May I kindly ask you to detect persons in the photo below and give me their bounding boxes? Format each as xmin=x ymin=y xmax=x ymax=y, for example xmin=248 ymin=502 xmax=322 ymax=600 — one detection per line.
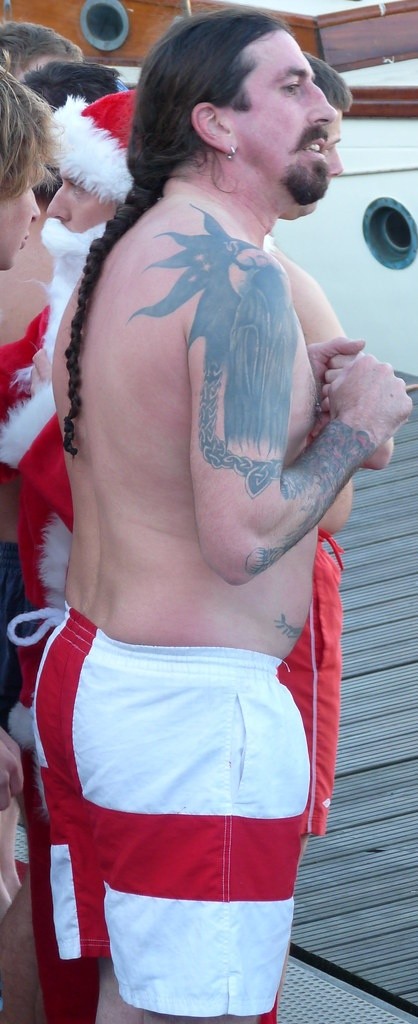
xmin=35 ymin=7 xmax=413 ymax=1024
xmin=0 ymin=23 xmax=396 ymax=1024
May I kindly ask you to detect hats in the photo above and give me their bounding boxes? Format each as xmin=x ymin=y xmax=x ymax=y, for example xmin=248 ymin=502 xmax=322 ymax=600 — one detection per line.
xmin=50 ymin=88 xmax=136 ymax=204
